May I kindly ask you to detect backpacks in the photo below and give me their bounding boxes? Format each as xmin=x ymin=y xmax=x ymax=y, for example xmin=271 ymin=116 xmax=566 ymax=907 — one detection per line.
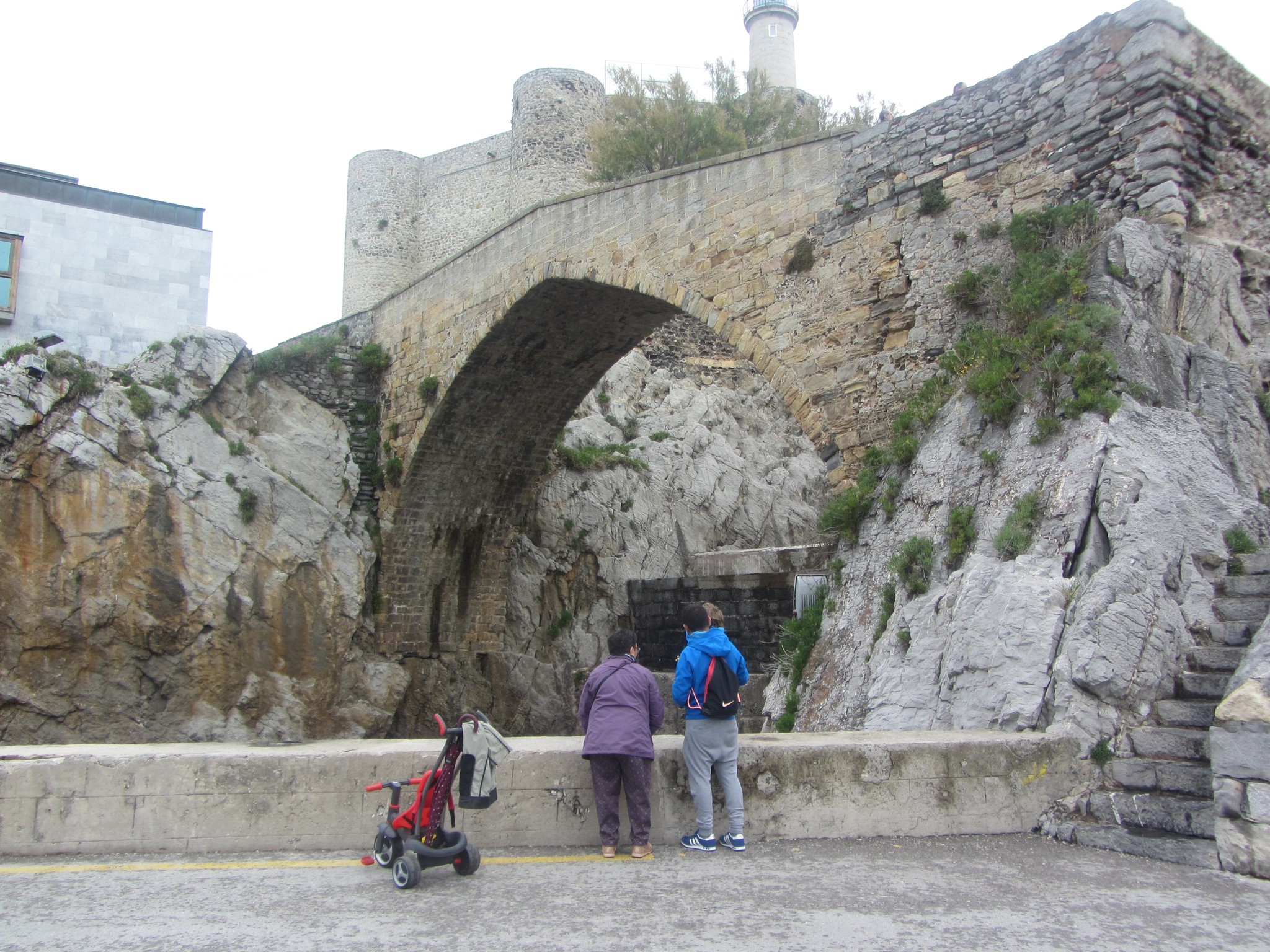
xmin=688 ymin=655 xmax=741 ymax=719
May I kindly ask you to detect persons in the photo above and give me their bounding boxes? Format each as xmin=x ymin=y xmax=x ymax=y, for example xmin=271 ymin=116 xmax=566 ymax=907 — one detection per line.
xmin=578 ymin=628 xmax=664 ymax=858
xmin=672 ymin=603 xmax=750 ymax=851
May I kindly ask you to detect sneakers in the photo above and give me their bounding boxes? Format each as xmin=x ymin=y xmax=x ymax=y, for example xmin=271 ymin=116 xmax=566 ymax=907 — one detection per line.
xmin=602 ymin=845 xmax=616 ymax=857
xmin=631 ymin=842 xmax=652 ymax=857
xmin=680 ymin=829 xmax=716 ymax=851
xmin=720 ymin=831 xmax=746 ymax=851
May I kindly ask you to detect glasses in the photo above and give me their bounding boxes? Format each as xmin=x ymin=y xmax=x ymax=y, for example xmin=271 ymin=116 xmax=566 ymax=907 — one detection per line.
xmin=630 ymin=647 xmax=640 ymax=653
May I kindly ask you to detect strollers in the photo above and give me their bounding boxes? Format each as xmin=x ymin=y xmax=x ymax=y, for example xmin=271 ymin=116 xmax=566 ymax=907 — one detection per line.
xmin=361 ymin=709 xmax=489 ymax=889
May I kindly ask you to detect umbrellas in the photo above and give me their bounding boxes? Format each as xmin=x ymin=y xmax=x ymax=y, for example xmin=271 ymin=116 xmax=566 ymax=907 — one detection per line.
xmin=424 ymin=714 xmax=478 ymax=847
